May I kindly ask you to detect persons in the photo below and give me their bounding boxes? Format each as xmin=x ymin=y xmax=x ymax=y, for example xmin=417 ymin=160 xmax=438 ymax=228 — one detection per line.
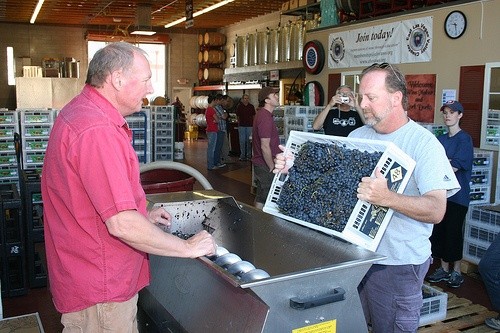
xmin=42 ymin=41 xmax=218 ymax=333
xmin=236 ymin=94 xmax=255 ymax=160
xmin=428 ymin=100 xmax=474 ymax=287
xmin=477 ymin=231 xmax=500 ymax=330
xmin=253 ymin=87 xmax=281 ymax=210
xmin=272 ymin=65 xmax=461 ymax=333
xmin=312 ymin=85 xmax=368 ymax=137
xmin=205 ymin=94 xmax=233 ymax=169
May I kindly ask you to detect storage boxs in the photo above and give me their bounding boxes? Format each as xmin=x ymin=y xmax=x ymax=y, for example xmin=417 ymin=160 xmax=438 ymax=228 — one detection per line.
xmin=271 ymin=105 xmax=326 ymax=145
xmin=417 ymin=285 xmax=448 ymax=327
xmin=261 ymin=130 xmax=415 ymax=253
xmin=413 ymin=122 xmax=500 ymax=265
xmin=125 ymin=104 xmax=175 ymax=163
xmin=1 ymin=109 xmax=61 ymax=195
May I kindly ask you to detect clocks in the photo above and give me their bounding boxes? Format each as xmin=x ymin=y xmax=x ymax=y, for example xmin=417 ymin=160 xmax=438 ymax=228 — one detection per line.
xmin=443 ymin=9 xmax=468 ymax=40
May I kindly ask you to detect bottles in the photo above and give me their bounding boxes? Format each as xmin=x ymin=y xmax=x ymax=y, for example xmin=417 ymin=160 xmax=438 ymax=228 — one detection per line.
xmin=235 ymin=18 xmax=320 ymax=65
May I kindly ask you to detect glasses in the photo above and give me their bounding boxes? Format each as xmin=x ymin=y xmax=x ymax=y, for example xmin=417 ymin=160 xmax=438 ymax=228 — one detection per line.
xmin=372 ymin=62 xmax=402 ymax=83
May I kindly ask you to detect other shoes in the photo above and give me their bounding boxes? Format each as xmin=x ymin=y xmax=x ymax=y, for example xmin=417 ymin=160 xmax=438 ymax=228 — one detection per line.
xmin=217 ymin=163 xmax=225 ymax=167
xmin=247 ymin=157 xmax=251 ymax=162
xmin=223 ymin=160 xmax=231 ymax=164
xmin=239 ymin=157 xmax=246 ymax=161
xmin=210 ymin=165 xmax=218 ymax=169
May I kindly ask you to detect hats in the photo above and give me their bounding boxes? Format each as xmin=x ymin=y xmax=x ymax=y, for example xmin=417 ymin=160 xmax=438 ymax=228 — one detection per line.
xmin=257 ymin=87 xmax=280 ymax=101
xmin=440 ymin=100 xmax=463 ymax=115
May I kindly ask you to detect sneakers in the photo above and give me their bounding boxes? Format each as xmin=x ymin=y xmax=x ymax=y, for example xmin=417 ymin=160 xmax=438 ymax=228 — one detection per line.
xmin=428 ymin=267 xmax=450 ymax=283
xmin=447 ymin=271 xmax=464 ymax=287
xmin=485 ymin=317 xmax=500 ymax=331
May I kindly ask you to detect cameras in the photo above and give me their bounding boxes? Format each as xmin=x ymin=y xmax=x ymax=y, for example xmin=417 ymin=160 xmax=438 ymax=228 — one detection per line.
xmin=338 ymin=97 xmax=349 ymax=104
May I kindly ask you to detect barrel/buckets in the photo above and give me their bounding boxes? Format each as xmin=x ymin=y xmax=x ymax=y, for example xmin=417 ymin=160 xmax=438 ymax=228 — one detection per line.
xmin=188 ymin=95 xmax=213 ymax=127
xmin=65 ymin=58 xmax=79 ymax=78
xmin=197 ymin=32 xmax=225 ymax=82
